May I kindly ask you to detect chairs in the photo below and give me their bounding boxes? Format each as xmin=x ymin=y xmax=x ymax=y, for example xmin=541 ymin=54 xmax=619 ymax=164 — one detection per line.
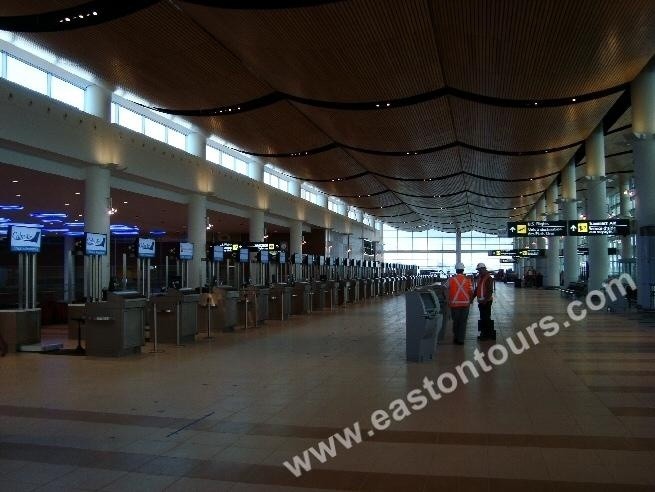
xmin=559 ymin=282 xmax=587 ymax=300
xmin=624 ymin=287 xmax=638 ymax=308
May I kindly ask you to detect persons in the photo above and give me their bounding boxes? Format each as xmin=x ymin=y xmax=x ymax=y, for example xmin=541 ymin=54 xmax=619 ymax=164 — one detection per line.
xmin=470 ymin=263 xmax=496 ymax=340
xmin=444 ymin=262 xmax=473 ymax=344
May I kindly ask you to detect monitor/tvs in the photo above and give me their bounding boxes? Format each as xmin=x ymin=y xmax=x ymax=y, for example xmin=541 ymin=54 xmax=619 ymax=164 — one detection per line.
xmin=307 ymin=254 xmax=313 ymax=264
xmin=294 ymin=253 xmax=301 ymax=264
xmin=279 ymin=252 xmax=286 ymax=264
xmin=213 ymin=245 xmax=224 ymax=262
xmin=179 ymin=242 xmax=194 ymax=260
xmin=239 ymin=248 xmax=249 ymax=263
xmin=9 ymin=225 xmax=43 ymax=254
xmin=319 ymin=255 xmax=412 ymax=270
xmin=260 ymin=250 xmax=269 ymax=263
xmin=136 ymin=237 xmax=157 ymax=258
xmin=85 ymin=232 xmax=107 ymax=255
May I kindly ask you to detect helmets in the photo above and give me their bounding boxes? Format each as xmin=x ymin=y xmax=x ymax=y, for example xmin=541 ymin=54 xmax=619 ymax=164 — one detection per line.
xmin=455 ymin=263 xmax=465 ymax=270
xmin=476 ymin=263 xmax=486 ymax=270
